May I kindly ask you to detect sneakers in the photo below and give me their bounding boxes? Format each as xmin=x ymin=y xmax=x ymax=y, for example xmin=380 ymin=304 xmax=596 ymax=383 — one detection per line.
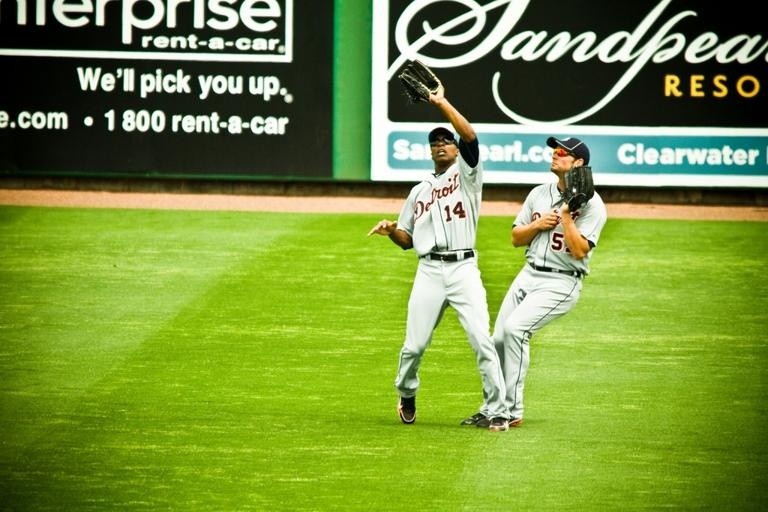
xmin=460 ymin=413 xmax=523 ymax=432
xmin=398 ymin=397 xmax=416 ymax=424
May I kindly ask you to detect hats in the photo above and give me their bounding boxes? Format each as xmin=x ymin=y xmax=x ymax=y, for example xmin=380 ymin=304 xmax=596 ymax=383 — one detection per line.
xmin=547 ymin=137 xmax=590 ymax=165
xmin=429 ymin=127 xmax=457 ymax=149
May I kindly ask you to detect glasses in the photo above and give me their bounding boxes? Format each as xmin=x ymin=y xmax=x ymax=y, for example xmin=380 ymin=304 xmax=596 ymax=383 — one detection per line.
xmin=553 ymin=146 xmax=577 ymax=158
xmin=430 ymin=137 xmax=453 ymax=146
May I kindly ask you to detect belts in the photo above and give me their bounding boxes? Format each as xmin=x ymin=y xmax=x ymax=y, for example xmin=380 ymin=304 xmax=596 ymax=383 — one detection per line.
xmin=529 ymin=263 xmax=581 ymax=277
xmin=419 ymin=251 xmax=474 ymax=261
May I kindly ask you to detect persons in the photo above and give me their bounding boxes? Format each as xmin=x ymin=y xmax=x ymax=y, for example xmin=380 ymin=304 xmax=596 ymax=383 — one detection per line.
xmin=368 ymin=60 xmax=510 ymax=431
xmin=461 ymin=136 xmax=607 ymax=428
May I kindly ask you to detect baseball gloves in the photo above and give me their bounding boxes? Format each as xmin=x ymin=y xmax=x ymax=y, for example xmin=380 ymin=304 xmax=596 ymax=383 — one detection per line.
xmin=561 ymin=166 xmax=593 ymax=212
xmin=398 ymin=59 xmax=439 ymax=102
